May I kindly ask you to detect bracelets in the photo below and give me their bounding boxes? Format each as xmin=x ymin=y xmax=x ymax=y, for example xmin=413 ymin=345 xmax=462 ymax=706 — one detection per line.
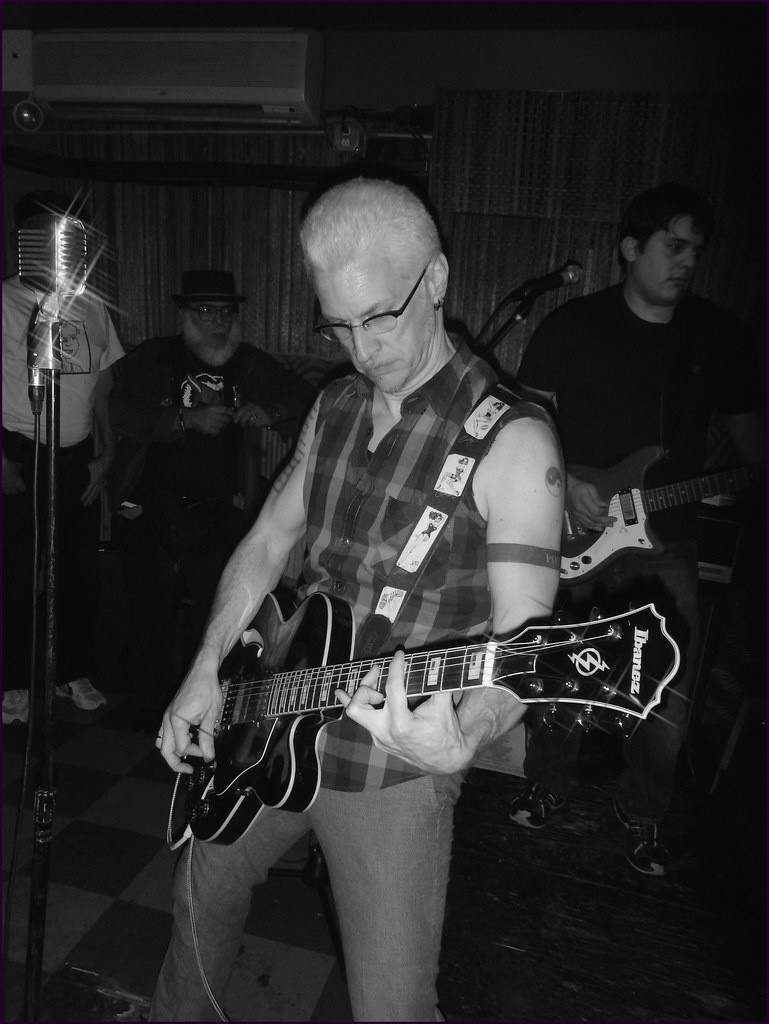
xmin=179 ymin=409 xmax=186 ymax=431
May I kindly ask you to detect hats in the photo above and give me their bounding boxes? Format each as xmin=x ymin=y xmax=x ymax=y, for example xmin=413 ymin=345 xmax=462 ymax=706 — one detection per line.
xmin=15 ymin=190 xmax=91 ymax=229
xmin=172 ymin=270 xmax=247 ymax=303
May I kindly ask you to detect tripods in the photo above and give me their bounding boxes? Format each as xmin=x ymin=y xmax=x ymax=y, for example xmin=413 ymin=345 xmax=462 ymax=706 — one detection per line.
xmin=268 ymin=840 xmax=344 ymax=983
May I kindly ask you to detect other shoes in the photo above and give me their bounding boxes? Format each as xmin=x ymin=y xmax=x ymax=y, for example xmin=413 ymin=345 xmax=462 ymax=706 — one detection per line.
xmin=134 ymin=705 xmax=161 ymax=734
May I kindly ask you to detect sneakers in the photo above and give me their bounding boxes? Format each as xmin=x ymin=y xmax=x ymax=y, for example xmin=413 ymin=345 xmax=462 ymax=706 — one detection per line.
xmin=508 ymin=782 xmax=568 ymax=830
xmin=56 ymin=677 xmax=107 ymax=711
xmin=612 ymin=793 xmax=670 ymax=876
xmin=2 ymin=689 xmax=29 ymax=726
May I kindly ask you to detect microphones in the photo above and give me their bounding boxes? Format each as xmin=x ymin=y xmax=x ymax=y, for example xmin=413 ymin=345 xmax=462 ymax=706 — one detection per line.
xmin=511 ymin=264 xmax=583 ymax=304
xmin=17 ymin=213 xmax=89 ymax=413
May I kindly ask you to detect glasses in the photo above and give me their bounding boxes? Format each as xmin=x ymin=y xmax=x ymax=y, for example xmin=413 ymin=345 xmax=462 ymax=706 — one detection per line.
xmin=179 ymin=304 xmax=239 ymax=322
xmin=312 ymin=247 xmax=442 ymax=343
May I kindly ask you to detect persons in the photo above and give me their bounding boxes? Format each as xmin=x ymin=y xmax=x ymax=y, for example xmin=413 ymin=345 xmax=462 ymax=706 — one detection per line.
xmin=151 ymin=175 xmax=567 ymax=1024
xmin=0 ymin=188 xmax=123 ymax=724
xmin=514 ymin=183 xmax=767 ymax=875
xmin=93 ymin=269 xmax=316 ymax=678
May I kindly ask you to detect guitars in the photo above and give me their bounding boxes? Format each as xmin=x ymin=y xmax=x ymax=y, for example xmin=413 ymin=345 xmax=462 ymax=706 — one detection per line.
xmin=557 ymin=444 xmax=769 ymax=589
xmin=187 ymin=585 xmax=682 ymax=849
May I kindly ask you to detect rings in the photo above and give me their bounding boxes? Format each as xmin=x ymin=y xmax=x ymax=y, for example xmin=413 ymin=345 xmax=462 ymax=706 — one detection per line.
xmin=248 ymin=414 xmax=256 ymax=422
xmin=158 ymin=735 xmax=162 ymax=738
